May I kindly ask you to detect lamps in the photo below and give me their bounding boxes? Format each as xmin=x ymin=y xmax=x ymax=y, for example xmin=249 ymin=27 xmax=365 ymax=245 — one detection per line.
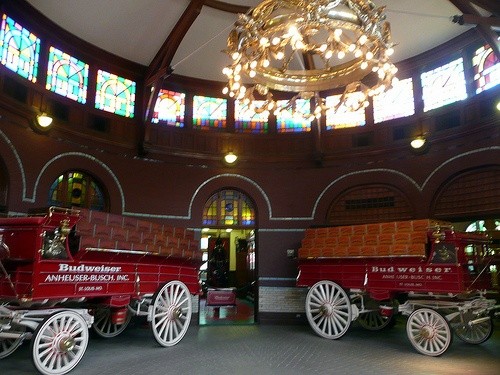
xmin=222 ymin=152 xmax=240 ymax=168
xmin=32 ymin=114 xmax=55 ymax=132
xmin=222 ymin=0 xmax=399 ymax=123
xmin=409 ymin=137 xmax=429 ymax=154
